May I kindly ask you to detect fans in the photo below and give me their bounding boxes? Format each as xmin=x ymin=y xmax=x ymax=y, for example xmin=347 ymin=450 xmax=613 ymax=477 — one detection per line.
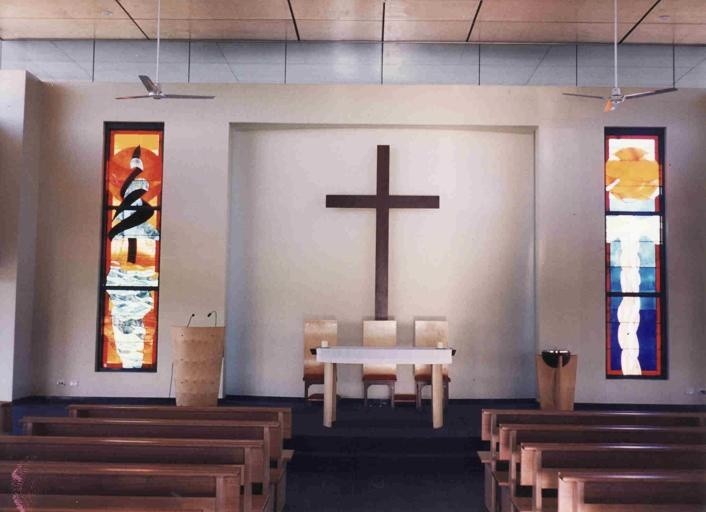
xmin=562 ymin=0 xmax=676 ymax=110
xmin=116 ymin=0 xmax=215 ymax=100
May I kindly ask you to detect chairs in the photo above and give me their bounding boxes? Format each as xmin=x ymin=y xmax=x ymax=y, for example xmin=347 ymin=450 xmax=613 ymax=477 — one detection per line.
xmin=303 ymin=319 xmax=338 ymax=404
xmin=414 ymin=320 xmax=451 ymax=406
xmin=361 ymin=320 xmax=397 ymax=407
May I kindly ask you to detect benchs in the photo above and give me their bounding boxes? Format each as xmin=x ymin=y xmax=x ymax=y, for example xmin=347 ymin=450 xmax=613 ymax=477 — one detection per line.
xmin=477 ymin=408 xmax=706 ymax=511
xmin=0 ymin=405 xmax=294 ymax=512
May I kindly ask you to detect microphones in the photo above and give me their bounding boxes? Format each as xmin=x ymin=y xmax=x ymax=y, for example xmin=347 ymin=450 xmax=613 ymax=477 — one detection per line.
xmin=208 ymin=310 xmax=217 ymax=327
xmin=187 ymin=313 xmax=195 ymax=327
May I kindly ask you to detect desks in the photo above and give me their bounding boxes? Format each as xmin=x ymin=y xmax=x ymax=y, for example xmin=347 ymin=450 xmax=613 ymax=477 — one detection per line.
xmin=317 ymin=346 xmax=452 ymax=428
